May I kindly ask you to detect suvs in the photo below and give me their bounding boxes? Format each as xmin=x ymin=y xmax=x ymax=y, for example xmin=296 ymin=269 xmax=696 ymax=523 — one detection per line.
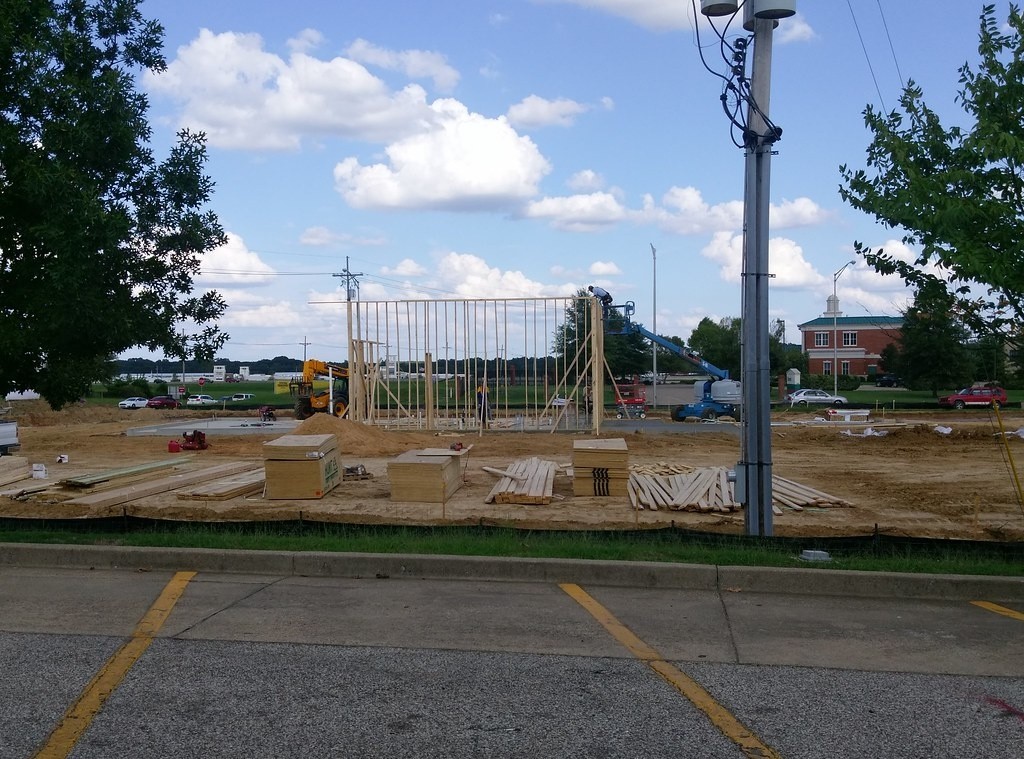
xmin=232 ymin=393 xmax=257 ymax=403
xmin=186 ymin=394 xmax=219 ymax=405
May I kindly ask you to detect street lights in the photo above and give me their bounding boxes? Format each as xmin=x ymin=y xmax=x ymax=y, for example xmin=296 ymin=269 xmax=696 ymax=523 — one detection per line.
xmin=834 ymin=260 xmax=857 ymax=398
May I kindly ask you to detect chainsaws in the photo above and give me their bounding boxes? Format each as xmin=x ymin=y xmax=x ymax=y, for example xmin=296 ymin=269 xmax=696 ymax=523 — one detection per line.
xmin=449 ymin=442 xmax=463 ymax=450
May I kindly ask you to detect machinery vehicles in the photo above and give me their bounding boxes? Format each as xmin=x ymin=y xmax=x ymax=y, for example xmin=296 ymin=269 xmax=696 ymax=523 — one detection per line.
xmin=602 ymin=299 xmax=742 ymax=423
xmin=288 ymin=359 xmax=370 ymax=420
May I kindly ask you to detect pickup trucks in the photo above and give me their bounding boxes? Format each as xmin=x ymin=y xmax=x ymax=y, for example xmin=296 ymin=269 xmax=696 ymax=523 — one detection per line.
xmin=0 ymin=419 xmax=21 ymax=456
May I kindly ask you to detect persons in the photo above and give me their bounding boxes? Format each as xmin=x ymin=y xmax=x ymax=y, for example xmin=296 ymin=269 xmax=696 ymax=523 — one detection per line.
xmin=588 ymin=285 xmax=611 ymax=331
xmin=583 ymin=382 xmax=593 ymax=415
xmin=477 ymin=377 xmax=491 ymax=429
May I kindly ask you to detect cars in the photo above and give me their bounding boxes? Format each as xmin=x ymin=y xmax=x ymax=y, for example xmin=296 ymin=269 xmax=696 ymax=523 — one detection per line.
xmin=786 ymin=389 xmax=848 ymax=405
xmin=226 ymin=378 xmax=239 ymax=383
xmin=875 ymin=373 xmax=905 ymax=388
xmin=148 ymin=395 xmax=182 ymax=409
xmin=218 ymin=395 xmax=232 ymax=403
xmin=939 ymin=385 xmax=1007 ymax=410
xmin=118 ymin=396 xmax=148 ymax=409
xmin=154 ymin=379 xmax=166 ymax=384
